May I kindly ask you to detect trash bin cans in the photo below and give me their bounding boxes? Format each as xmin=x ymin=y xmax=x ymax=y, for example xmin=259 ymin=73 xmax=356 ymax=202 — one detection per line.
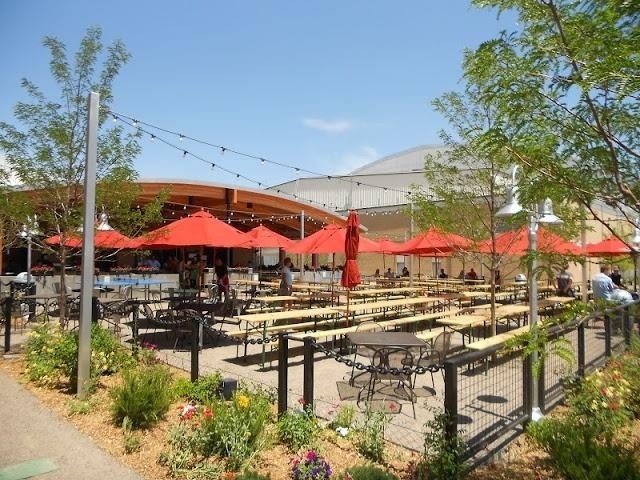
xmin=515 ymin=274 xmax=527 ymax=282
xmin=10 ymin=272 xmax=36 ymax=320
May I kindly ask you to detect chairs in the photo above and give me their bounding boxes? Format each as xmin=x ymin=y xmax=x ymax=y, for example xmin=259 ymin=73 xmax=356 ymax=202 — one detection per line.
xmin=0 ymin=263 xmax=640 ymax=419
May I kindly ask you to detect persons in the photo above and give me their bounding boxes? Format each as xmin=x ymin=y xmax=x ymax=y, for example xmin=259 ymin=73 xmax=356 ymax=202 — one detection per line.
xmin=551 ymin=259 xmax=576 ymax=298
xmin=609 ymin=266 xmax=629 ymax=290
xmin=456 ymin=270 xmax=465 ymax=279
xmin=608 ymin=287 xmax=638 ymax=303
xmin=593 ymin=265 xmax=614 ymax=300
xmin=143 ymin=253 xmax=208 ymax=289
xmin=374 ymin=268 xmax=381 ymax=278
xmin=466 ymin=267 xmax=477 ymax=285
xmin=439 ymin=268 xmax=448 ymax=278
xmin=398 ymin=266 xmax=407 ymax=277
xmin=384 ymin=268 xmax=393 ymax=279
xmin=212 ymin=256 xmax=230 ymax=303
xmin=279 ymin=257 xmax=294 ymax=312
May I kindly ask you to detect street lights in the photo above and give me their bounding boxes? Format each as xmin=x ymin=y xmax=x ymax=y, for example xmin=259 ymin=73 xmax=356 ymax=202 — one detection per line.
xmin=631 ymin=217 xmax=640 ymax=244
xmin=19 ymin=212 xmax=41 ymax=284
xmin=495 ymin=164 xmax=565 ymax=422
xmin=75 ymin=205 xmax=115 ymax=233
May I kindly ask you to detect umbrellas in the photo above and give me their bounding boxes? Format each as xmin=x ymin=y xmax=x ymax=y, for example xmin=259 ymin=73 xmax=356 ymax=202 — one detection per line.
xmin=467 ymin=223 xmax=588 ymax=293
xmin=44 ymin=214 xmax=129 ymax=252
xmin=365 ymin=236 xmax=400 ymax=267
xmin=241 ymin=222 xmax=292 ymax=249
xmin=288 ymin=221 xmax=383 ymax=275
xmin=125 ymin=210 xmax=262 ymax=292
xmin=339 ymin=209 xmax=361 ymax=329
xmin=392 ymin=226 xmax=475 ymax=282
xmin=586 ymin=235 xmax=640 ymax=255
